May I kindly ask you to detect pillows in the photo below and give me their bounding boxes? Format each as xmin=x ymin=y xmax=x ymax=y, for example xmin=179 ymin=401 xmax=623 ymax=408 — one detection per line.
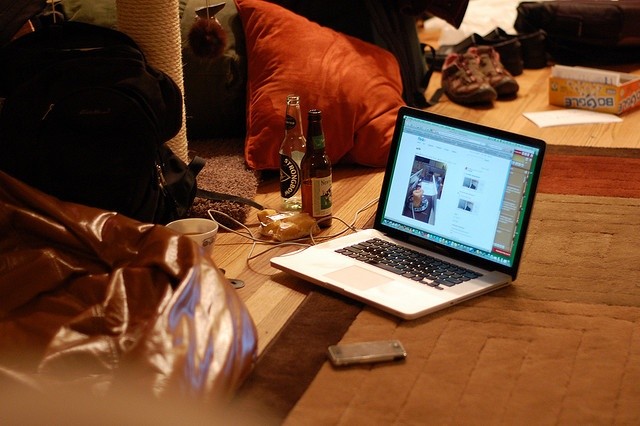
xmin=233 ymin=0 xmax=409 ymax=168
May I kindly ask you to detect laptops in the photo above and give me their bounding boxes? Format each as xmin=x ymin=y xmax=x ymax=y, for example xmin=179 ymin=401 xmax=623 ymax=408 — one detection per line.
xmin=269 ymin=105 xmax=547 ymax=318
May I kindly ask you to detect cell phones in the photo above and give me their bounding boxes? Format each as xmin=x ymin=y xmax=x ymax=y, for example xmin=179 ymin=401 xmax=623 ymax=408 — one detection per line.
xmin=327 ymin=338 xmax=407 ymax=370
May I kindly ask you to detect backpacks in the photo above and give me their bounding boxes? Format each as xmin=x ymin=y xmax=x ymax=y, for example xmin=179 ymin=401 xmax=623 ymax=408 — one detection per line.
xmin=1 ymin=1 xmax=264 ymax=227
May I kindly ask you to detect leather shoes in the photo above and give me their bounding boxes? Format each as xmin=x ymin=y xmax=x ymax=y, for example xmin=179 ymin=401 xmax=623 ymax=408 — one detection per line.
xmin=425 ymin=33 xmax=523 ymax=76
xmin=481 ymin=27 xmax=550 ymax=68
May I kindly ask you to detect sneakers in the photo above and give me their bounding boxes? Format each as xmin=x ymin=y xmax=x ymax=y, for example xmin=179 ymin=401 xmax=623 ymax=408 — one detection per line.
xmin=441 ymin=52 xmax=497 ymax=105
xmin=466 ymin=46 xmax=519 ymax=97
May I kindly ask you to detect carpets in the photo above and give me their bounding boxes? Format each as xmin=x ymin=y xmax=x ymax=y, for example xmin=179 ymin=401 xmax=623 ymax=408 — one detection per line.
xmin=225 ymin=142 xmax=640 ymax=426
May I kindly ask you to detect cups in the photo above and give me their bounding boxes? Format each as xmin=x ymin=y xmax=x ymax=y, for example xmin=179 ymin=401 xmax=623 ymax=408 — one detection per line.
xmin=164 ymin=218 xmax=219 ymax=263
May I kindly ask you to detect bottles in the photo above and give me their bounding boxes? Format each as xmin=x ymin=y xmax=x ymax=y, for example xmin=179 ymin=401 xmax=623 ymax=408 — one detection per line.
xmin=300 ymin=108 xmax=332 ymax=228
xmin=279 ymin=94 xmax=307 ymax=211
